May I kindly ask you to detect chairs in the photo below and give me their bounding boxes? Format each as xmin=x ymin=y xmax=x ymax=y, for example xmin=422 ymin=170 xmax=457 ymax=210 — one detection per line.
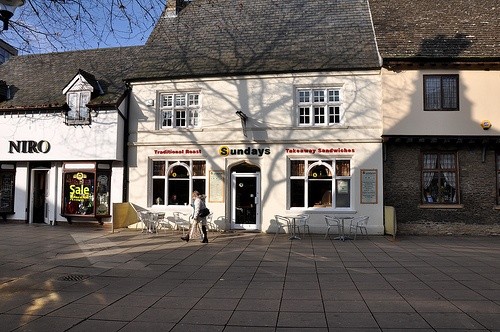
xmin=138 ymin=210 xmax=216 ymax=240
xmin=274 ymin=214 xmax=370 ymax=240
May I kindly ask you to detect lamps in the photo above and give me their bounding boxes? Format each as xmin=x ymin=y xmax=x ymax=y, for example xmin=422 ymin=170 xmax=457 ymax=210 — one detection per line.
xmin=236 ymin=111 xmax=248 ymax=121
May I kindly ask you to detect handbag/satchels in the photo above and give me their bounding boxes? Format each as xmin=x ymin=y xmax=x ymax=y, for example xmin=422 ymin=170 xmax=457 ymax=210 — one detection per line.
xmin=199 ymin=208 xmax=210 ymax=218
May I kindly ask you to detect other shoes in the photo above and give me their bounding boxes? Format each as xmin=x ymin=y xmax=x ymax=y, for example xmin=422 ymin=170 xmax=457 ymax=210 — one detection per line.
xmin=200 ymin=239 xmax=208 ymax=243
xmin=181 ymin=237 xmax=189 ymax=242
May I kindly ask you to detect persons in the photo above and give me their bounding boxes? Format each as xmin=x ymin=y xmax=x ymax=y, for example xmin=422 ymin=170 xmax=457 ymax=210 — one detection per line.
xmin=181 ymin=191 xmax=208 ymax=243
xmin=169 ymin=195 xmax=179 ymax=205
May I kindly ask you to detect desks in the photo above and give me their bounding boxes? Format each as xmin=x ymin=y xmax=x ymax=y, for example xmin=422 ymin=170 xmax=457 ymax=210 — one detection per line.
xmin=336 ymin=216 xmax=353 ymax=240
xmin=282 ymin=215 xmax=301 ymax=239
